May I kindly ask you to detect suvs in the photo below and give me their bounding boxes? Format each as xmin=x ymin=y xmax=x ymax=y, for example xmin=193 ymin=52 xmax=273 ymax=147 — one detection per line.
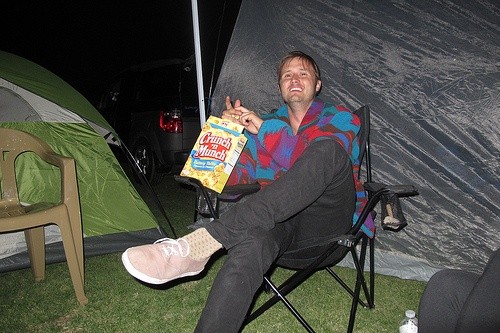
xmin=96 ymin=42 xmax=211 ymax=188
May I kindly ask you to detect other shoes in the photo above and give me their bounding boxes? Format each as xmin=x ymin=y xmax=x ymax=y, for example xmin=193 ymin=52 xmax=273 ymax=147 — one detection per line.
xmin=122 ymin=237 xmax=210 ymax=284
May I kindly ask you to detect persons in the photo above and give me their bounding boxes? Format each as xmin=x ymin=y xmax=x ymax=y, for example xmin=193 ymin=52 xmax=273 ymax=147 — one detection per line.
xmin=416 ymin=248 xmax=500 ymax=333
xmin=121 ymin=49 xmax=377 ymax=332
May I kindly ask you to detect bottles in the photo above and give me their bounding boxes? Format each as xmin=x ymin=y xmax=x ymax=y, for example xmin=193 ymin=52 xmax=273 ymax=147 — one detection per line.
xmin=199 ymin=192 xmax=217 ymax=213
xmin=399 ymin=310 xmax=418 ymax=333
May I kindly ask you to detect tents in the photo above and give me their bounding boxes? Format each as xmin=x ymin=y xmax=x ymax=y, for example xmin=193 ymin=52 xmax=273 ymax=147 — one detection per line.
xmin=0 ymin=49 xmax=170 ymax=275
xmin=194 ymin=0 xmax=500 ymax=286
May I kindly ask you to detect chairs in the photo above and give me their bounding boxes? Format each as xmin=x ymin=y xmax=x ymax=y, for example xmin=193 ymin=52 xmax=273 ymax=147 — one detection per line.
xmin=0 ymin=128 xmax=88 ymax=306
xmin=176 ymin=105 xmax=419 ymax=333
